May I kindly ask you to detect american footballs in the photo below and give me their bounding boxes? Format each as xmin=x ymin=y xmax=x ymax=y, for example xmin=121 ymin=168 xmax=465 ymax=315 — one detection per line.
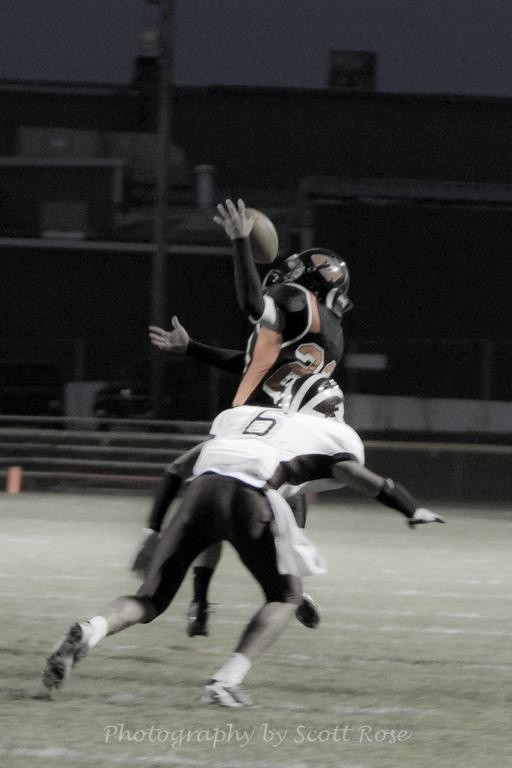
xmin=245 ymin=210 xmax=278 ymax=262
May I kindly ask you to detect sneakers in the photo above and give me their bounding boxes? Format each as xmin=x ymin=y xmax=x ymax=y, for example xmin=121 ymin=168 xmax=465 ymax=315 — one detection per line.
xmin=202 ymin=677 xmax=260 ymax=710
xmin=188 ymin=601 xmax=209 ymax=637
xmin=292 ymin=594 xmax=319 ymax=629
xmin=42 ymin=621 xmax=93 ymax=691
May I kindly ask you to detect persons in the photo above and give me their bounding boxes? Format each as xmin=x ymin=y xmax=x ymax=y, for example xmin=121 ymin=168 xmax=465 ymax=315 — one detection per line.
xmin=42 ymin=374 xmax=448 ymax=709
xmin=147 ymin=199 xmax=354 ymax=636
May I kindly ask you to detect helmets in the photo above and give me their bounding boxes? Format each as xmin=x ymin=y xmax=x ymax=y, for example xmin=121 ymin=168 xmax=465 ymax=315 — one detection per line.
xmin=283 ymin=248 xmax=350 ymax=302
xmin=281 ymin=372 xmax=346 ymax=424
